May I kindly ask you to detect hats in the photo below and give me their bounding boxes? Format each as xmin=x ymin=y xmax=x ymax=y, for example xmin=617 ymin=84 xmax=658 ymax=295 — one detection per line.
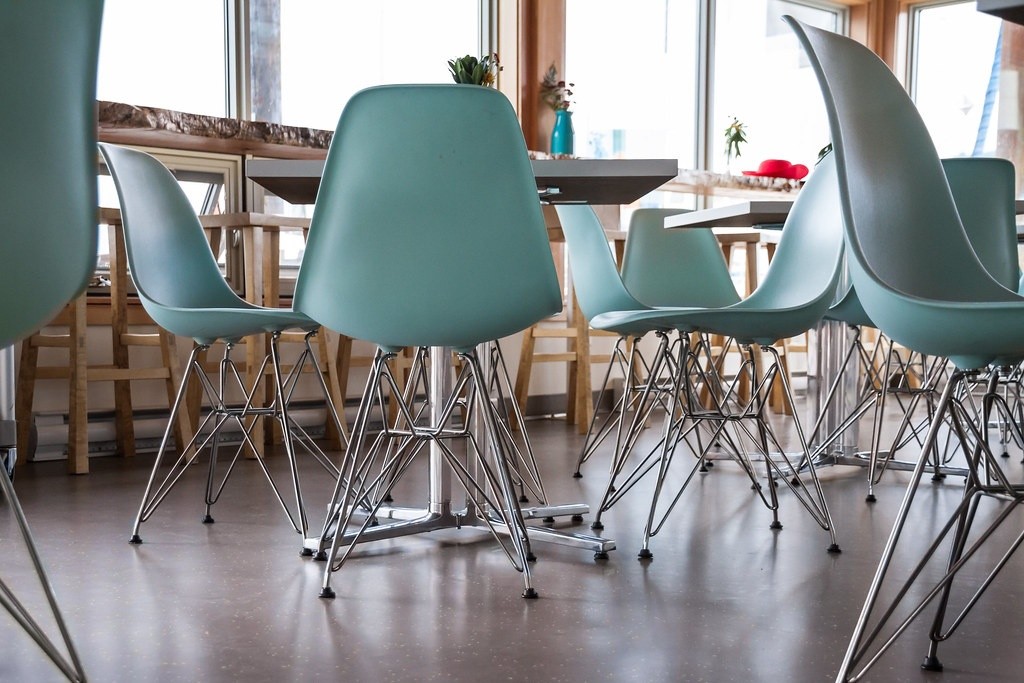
xmin=741 ymin=159 xmax=810 ymax=179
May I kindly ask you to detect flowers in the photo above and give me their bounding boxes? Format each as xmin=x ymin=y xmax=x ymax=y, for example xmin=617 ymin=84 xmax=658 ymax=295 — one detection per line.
xmin=722 ymin=117 xmax=748 ymax=168
xmin=536 ymin=60 xmax=576 ymax=112
xmin=447 ymin=53 xmax=504 ymax=88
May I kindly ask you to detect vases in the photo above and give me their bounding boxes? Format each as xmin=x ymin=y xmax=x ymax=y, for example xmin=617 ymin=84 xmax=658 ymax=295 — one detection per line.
xmin=551 ymin=111 xmax=573 ymax=155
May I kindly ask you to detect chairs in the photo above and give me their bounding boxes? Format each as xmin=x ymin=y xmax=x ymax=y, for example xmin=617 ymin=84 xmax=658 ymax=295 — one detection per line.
xmin=0 ymin=0 xmax=1024 ymax=683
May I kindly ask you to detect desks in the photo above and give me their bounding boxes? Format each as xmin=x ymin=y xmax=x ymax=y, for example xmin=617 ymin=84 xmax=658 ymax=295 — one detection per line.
xmin=95 ymin=97 xmax=807 ymax=199
xmin=245 ymin=159 xmax=678 ymax=559
xmin=664 ymin=202 xmax=1024 ymax=489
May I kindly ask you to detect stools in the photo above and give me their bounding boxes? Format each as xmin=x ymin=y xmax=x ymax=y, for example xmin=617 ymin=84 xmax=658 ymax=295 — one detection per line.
xmin=509 ymin=227 xmax=651 ymax=436
xmin=678 ymin=232 xmax=765 ymax=416
xmin=185 ymin=213 xmax=350 ymax=460
xmin=16 ymin=207 xmax=199 ymax=476
